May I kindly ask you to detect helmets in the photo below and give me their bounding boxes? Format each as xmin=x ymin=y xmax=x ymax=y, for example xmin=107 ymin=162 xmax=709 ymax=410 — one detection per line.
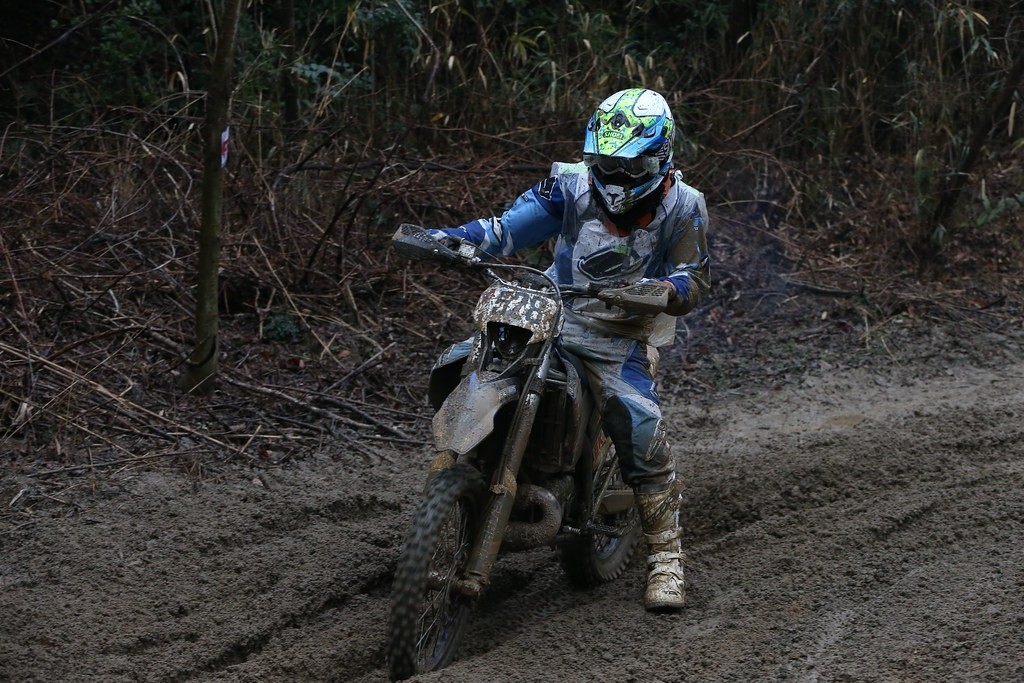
xmin=583 ymin=89 xmax=676 ymax=214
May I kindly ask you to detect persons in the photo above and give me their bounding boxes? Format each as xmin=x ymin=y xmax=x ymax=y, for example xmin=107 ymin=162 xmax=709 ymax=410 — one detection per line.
xmin=424 ymin=88 xmax=711 ymax=611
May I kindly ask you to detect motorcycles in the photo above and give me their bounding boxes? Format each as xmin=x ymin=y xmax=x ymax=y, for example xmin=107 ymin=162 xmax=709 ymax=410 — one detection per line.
xmin=385 ymin=225 xmax=674 ymax=682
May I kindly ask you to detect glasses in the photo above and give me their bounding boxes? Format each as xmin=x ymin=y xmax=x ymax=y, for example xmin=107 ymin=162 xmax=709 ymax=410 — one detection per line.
xmin=595 ymin=155 xmax=650 ymax=178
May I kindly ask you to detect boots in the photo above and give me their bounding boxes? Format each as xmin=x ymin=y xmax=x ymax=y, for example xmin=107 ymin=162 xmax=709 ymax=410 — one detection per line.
xmin=634 ymin=474 xmax=685 ymax=610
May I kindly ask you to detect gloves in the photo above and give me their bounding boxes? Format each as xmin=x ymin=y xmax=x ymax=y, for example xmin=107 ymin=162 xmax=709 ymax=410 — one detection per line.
xmin=638 ymin=277 xmax=661 ymax=286
xmin=414 ymin=227 xmax=446 ymax=246
